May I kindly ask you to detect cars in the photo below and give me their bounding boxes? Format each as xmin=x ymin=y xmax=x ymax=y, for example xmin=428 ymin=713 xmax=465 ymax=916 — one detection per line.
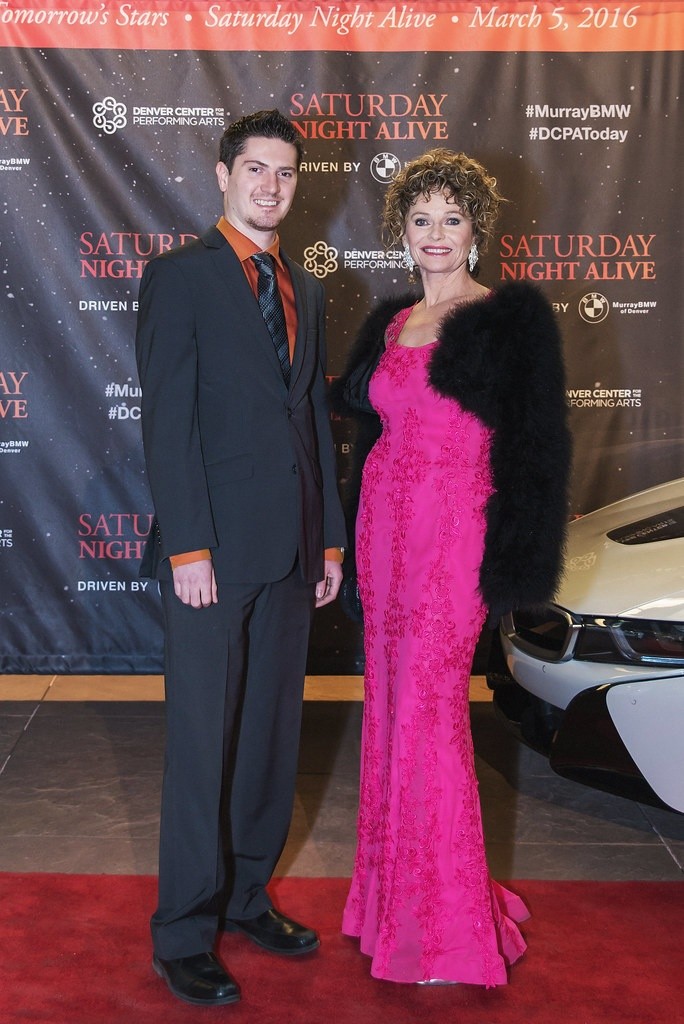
xmin=485 ymin=477 xmax=684 ymax=818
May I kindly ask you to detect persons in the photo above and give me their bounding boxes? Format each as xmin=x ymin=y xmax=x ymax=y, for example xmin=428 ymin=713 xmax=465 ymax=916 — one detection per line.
xmin=328 ymin=148 xmax=573 ymax=989
xmin=135 ymin=110 xmax=349 ymax=1006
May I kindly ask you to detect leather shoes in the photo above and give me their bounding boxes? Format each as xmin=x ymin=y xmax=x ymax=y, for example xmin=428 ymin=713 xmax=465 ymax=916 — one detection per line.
xmin=152 ymin=951 xmax=241 ymax=1006
xmin=219 ymin=908 xmax=320 ymax=956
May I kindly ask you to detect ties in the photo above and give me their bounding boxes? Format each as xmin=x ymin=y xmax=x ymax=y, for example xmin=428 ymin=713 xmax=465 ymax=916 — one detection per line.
xmin=251 ymin=252 xmax=291 ymax=393
